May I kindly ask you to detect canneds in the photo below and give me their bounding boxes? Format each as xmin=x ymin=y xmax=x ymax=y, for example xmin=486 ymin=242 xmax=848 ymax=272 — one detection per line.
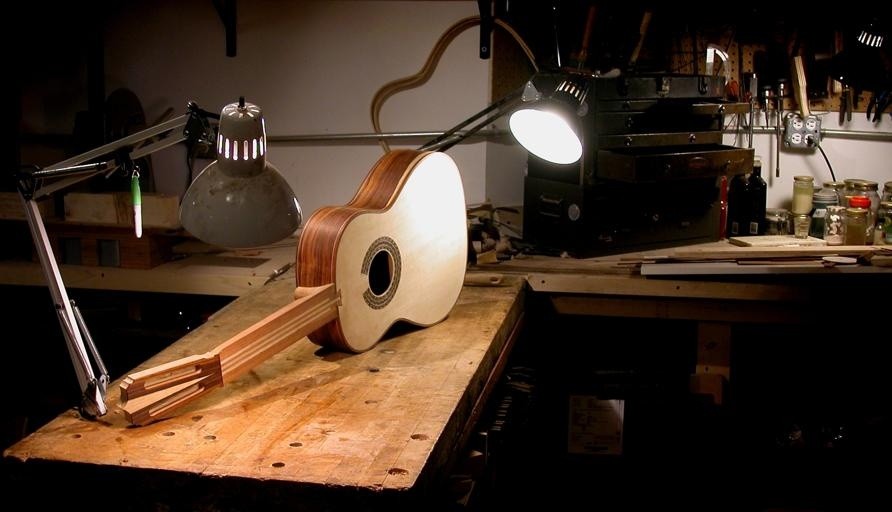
xmin=791 ymin=174 xmax=892 ymax=246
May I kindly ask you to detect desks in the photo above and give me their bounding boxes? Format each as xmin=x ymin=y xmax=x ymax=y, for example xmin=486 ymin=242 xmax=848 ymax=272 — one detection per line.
xmin=1 ymin=235 xmax=888 ymax=491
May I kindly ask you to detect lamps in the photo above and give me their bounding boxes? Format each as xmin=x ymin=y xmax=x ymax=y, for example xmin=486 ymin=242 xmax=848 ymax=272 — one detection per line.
xmin=825 ymin=20 xmax=892 ymax=93
xmin=18 ymin=95 xmax=304 ymax=421
xmin=418 ymin=69 xmax=591 ymax=165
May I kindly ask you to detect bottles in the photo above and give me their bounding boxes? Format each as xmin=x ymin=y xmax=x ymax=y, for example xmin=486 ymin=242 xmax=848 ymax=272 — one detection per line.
xmin=794 ymin=215 xmax=811 ymax=240
xmin=721 ymin=159 xmax=767 ymax=237
xmin=767 ymin=208 xmax=790 ymax=235
xmin=812 ymin=179 xmax=892 ymax=246
